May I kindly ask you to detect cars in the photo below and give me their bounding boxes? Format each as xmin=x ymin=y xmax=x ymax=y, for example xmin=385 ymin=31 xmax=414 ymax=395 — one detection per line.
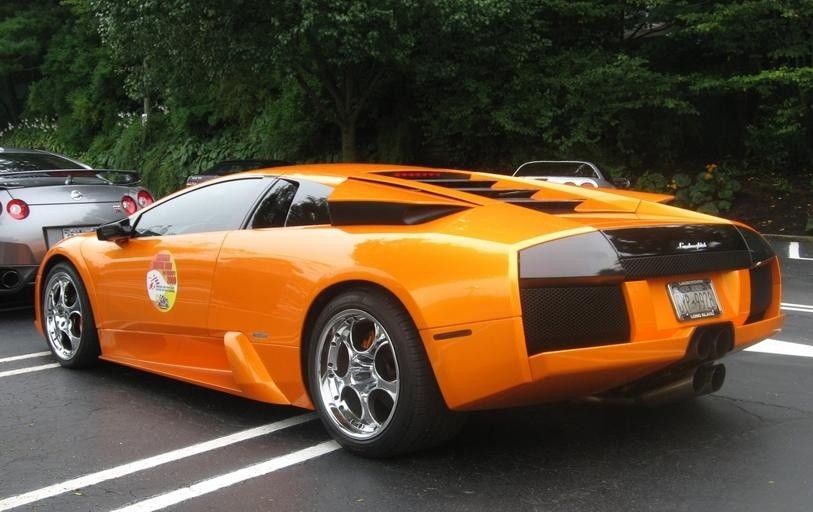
xmin=184 ymin=160 xmax=292 ymax=193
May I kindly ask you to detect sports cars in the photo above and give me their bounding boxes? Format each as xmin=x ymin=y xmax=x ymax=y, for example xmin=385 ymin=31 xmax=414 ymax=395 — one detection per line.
xmin=0 ymin=145 xmax=156 ymax=295
xmin=33 ymin=158 xmax=785 ymax=457
xmin=511 ymin=160 xmax=628 ymax=192
